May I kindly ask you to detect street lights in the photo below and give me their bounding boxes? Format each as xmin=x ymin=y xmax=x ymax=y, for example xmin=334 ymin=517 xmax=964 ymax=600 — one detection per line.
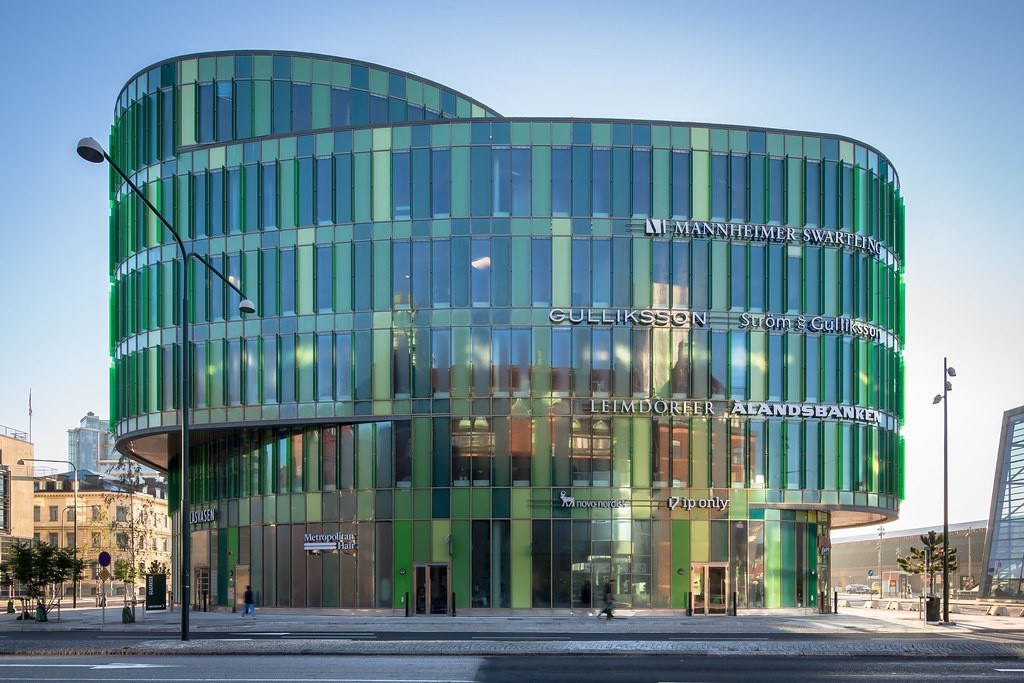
xmin=929 ymin=356 xmax=956 ymax=627
xmin=61 ymin=505 xmax=102 ymax=600
xmin=77 ymin=134 xmax=258 ymax=642
xmin=876 ymin=524 xmax=887 ymax=600
xmin=964 ymin=526 xmax=976 ymax=589
xmin=17 ymin=457 xmax=78 ymax=609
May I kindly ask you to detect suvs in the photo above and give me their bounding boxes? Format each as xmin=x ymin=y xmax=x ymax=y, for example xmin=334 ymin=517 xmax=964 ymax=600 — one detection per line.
xmin=872 ymin=582 xmax=880 ymax=594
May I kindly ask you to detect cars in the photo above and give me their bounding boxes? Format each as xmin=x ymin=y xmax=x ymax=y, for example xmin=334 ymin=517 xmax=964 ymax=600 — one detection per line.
xmin=847 ymin=584 xmax=871 ymax=594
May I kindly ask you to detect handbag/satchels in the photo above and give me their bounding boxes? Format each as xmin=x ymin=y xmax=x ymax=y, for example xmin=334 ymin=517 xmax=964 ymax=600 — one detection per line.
xmin=601 ymin=604 xmax=615 ymax=614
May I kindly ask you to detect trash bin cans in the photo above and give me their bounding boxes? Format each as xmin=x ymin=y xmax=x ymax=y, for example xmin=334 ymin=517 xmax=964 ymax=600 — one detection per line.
xmin=926 ymin=594 xmax=941 ymax=622
xmin=122 ymin=606 xmax=133 ymax=624
xmin=7 ymin=598 xmax=15 ymax=613
xmin=36 ymin=604 xmax=48 ymax=622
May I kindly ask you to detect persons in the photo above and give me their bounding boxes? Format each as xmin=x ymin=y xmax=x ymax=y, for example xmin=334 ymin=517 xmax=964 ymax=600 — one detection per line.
xmin=240 ymin=585 xmax=256 ymax=619
xmin=995 ymin=585 xmax=1002 ymax=597
xmin=604 ymin=579 xmax=615 ymax=620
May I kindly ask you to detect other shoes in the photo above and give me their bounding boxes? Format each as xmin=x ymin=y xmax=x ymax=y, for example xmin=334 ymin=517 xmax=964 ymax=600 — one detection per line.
xmin=606 ymin=616 xmax=612 ymax=620
xmin=240 ymin=616 xmax=245 ymax=619
xmin=251 ymin=616 xmax=256 ymax=620
